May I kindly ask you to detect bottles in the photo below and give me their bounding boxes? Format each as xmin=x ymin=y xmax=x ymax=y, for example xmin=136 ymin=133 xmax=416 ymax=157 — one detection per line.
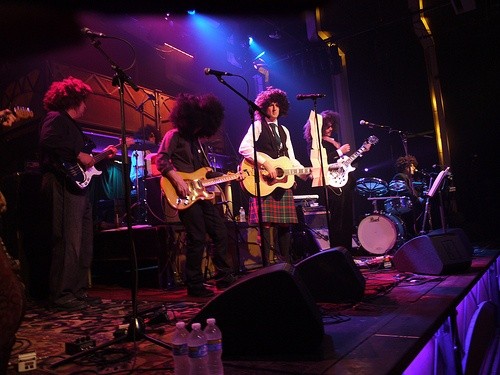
xmin=203 ymin=317 xmax=225 ymax=375
xmin=173 ymin=322 xmax=191 ymax=375
xmin=188 ymin=323 xmax=211 ymax=375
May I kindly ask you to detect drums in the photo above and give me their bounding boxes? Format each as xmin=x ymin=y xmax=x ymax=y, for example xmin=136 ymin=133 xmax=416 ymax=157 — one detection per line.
xmin=412 ymin=181 xmax=427 ymax=196
xmin=357 ymin=213 xmax=404 ymax=255
xmin=384 ymin=196 xmax=413 ymax=214
xmin=388 ymin=178 xmax=406 ymax=191
xmin=355 ymin=176 xmax=389 ymax=196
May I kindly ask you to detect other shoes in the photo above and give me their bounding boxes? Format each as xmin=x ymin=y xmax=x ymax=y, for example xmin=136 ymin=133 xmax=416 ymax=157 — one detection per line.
xmin=47 ymin=293 xmax=102 ymax=312
xmin=216 ymin=279 xmax=231 ymax=289
xmin=187 ymin=285 xmax=214 ymax=298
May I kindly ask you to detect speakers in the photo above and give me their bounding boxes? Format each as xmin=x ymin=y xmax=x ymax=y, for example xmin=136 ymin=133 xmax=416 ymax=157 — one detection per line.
xmin=186 ymin=262 xmax=325 ymax=362
xmin=294 ymin=206 xmax=331 ymax=255
xmin=392 ymin=228 xmax=473 ymax=276
xmin=294 ymin=246 xmax=367 ymax=304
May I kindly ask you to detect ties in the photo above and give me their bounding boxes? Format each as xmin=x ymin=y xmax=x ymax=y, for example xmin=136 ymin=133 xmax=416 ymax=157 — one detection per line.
xmin=269 ymin=123 xmax=281 ymax=149
xmin=191 ymin=138 xmax=201 ymax=171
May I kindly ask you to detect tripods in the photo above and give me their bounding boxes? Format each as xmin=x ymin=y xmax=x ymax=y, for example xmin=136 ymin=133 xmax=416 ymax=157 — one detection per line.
xmin=49 ymin=37 xmax=176 ymax=372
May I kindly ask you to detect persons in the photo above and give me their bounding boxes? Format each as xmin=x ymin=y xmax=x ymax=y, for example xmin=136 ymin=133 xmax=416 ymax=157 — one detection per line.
xmin=156 ymin=92 xmax=246 ymax=297
xmin=391 ymin=156 xmax=427 ymax=220
xmin=0 ymin=78 xmax=155 ymax=309
xmin=303 ymin=110 xmax=371 ymax=247
xmin=239 ymin=87 xmax=312 ymax=264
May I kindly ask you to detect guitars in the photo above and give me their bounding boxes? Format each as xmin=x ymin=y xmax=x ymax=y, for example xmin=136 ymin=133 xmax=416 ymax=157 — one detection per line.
xmin=237 ymin=151 xmax=313 ymax=196
xmin=159 ymin=166 xmax=250 ymax=210
xmin=327 ymin=134 xmax=380 ymax=188
xmin=52 ymin=140 xmax=124 ymax=196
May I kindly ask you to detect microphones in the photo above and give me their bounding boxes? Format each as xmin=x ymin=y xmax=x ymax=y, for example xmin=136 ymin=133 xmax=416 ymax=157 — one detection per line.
xmin=204 ymin=68 xmax=232 ymax=76
xmin=360 ymin=120 xmax=384 ymax=128
xmin=296 ymin=93 xmax=326 ymax=100
xmin=81 ymin=27 xmax=105 ymax=38
